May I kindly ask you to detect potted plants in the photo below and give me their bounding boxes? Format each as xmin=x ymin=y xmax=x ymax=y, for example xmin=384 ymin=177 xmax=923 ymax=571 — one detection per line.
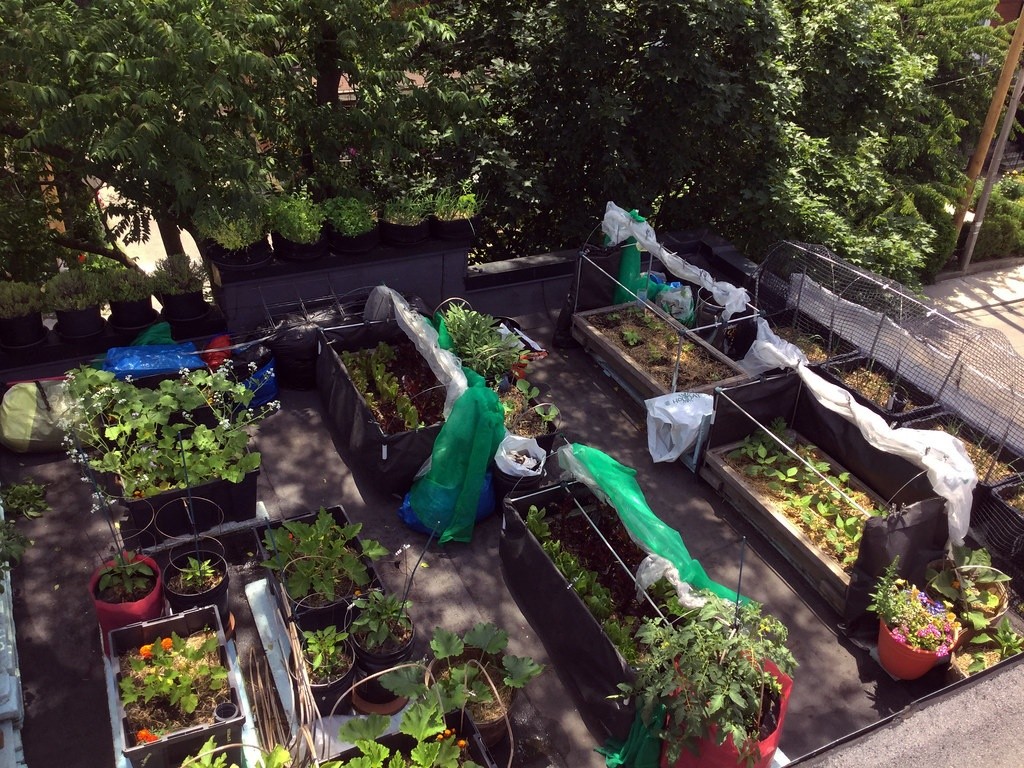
xmin=90 ymin=298 xmax=1024 ymax=767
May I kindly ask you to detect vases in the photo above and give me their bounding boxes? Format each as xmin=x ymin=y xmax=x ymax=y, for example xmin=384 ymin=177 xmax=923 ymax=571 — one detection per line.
xmin=110 ymin=442 xmax=261 ymax=546
xmin=102 ymin=371 xmax=229 ymax=446
xmin=876 ymin=615 xmax=957 ymax=678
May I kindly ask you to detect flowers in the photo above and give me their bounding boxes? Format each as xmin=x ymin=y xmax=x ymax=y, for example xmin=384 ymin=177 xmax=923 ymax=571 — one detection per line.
xmin=867 ymin=558 xmax=960 ymax=656
xmin=54 ymin=348 xmax=283 ymax=515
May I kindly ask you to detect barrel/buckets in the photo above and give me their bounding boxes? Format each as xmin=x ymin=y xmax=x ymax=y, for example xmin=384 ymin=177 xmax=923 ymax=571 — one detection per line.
xmin=693 ymin=282 xmax=756 ymax=358
xmin=490 ymin=460 xmax=548 ymax=519
